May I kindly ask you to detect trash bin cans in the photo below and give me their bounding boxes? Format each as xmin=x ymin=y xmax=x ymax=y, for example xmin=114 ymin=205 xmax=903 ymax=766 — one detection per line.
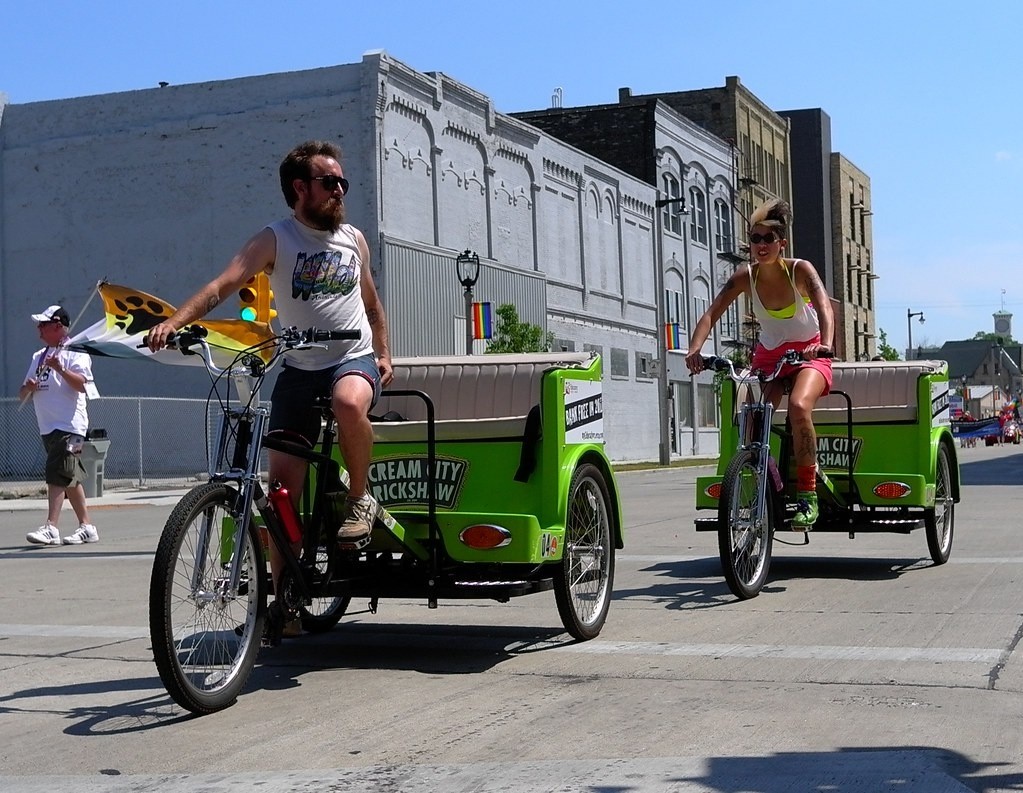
xmin=75 ymin=428 xmax=111 ymax=497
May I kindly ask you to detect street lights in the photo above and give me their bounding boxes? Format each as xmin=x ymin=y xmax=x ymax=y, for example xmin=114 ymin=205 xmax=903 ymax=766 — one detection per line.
xmin=962 ymin=374 xmax=967 ymax=413
xmin=908 ymin=308 xmax=925 ymax=359
xmin=456 ymin=249 xmax=480 ymax=355
xmin=1005 ymin=384 xmax=1010 ymax=402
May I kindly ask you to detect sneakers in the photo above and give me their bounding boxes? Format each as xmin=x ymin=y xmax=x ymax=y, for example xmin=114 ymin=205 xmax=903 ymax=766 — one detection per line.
xmin=27 ymin=524 xmax=62 ymax=545
xmin=792 ymin=491 xmax=821 ymax=526
xmin=63 ymin=523 xmax=101 ymax=547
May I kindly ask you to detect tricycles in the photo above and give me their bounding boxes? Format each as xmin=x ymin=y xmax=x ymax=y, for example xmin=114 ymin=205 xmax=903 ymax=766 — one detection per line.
xmin=137 ymin=324 xmax=625 ymax=715
xmin=686 ymin=348 xmax=960 ymax=599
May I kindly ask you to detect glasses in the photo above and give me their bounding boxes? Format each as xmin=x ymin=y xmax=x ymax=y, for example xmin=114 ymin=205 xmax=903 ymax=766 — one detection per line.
xmin=747 ymin=233 xmax=779 ymax=246
xmin=306 ymin=173 xmax=350 ymax=195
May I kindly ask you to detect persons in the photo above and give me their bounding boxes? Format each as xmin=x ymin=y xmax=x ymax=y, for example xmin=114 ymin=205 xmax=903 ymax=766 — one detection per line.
xmin=147 ymin=141 xmax=394 ymax=639
xmin=19 ymin=305 xmax=99 ymax=545
xmin=957 ymin=408 xmax=1022 ymax=448
xmin=685 ymin=199 xmax=834 ymax=532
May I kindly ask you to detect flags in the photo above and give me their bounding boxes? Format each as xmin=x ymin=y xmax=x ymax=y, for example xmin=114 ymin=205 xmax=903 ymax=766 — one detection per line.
xmin=64 ymin=280 xmax=276 ymax=369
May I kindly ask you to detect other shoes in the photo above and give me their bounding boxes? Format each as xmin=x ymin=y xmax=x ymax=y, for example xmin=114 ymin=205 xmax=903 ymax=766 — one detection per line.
xmin=235 ymin=621 xmax=287 ymax=642
xmin=337 ymin=493 xmax=381 ymax=546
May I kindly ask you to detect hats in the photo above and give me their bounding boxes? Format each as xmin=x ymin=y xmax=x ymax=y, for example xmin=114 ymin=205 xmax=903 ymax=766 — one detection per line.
xmin=30 ymin=305 xmax=74 ymax=329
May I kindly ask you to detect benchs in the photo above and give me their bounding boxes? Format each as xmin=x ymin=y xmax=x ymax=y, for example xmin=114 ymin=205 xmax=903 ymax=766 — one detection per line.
xmin=732 ymin=361 xmax=947 ymax=425
xmin=271 ymin=345 xmax=604 ymax=443
